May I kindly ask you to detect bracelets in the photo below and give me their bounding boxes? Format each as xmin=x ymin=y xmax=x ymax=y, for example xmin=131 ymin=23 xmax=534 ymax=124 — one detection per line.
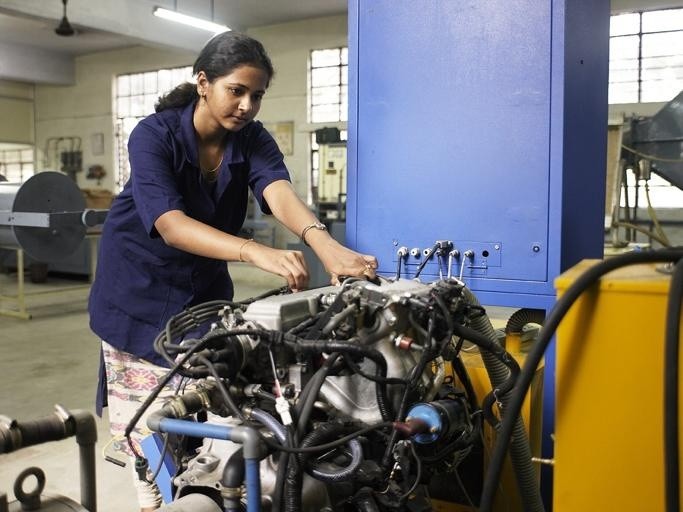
xmin=239 ymin=239 xmax=256 ymax=263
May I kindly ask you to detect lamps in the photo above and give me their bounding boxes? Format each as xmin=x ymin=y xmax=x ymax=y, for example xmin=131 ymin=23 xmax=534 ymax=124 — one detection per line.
xmin=152 ymin=0 xmax=233 ymax=40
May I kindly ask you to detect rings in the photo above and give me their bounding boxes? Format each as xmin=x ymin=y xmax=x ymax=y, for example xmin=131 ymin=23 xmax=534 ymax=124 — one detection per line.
xmin=365 ymin=264 xmax=371 ymax=269
xmin=361 ymin=268 xmax=369 ymax=275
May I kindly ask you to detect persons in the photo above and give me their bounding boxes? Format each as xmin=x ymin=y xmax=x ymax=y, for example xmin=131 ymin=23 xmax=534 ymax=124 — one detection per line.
xmin=87 ymin=31 xmax=378 ymax=512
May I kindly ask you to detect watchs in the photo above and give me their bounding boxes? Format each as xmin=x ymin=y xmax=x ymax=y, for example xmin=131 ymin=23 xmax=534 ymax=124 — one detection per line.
xmin=301 ymin=222 xmax=328 ymax=247
